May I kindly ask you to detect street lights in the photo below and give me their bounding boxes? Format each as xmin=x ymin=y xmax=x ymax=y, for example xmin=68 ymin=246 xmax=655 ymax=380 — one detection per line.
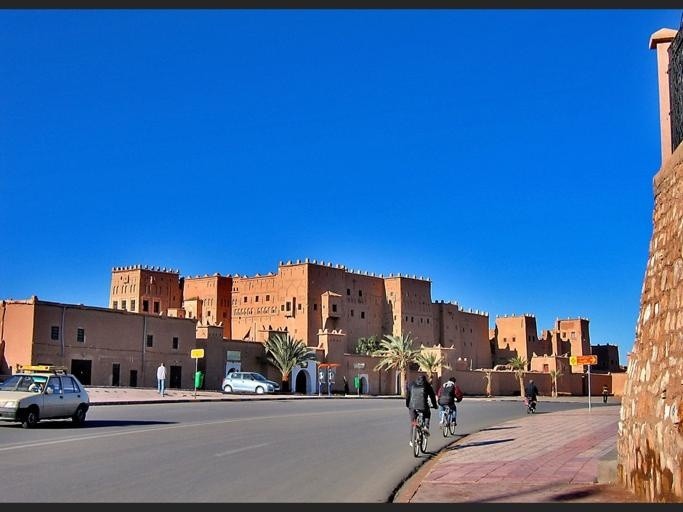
xmin=457 ymin=356 xmax=473 ymax=371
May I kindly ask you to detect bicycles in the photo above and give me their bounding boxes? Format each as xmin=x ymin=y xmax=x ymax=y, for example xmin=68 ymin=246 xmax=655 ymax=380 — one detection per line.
xmin=440 ymin=400 xmax=457 ymax=437
xmin=412 ymin=407 xmax=435 ymax=457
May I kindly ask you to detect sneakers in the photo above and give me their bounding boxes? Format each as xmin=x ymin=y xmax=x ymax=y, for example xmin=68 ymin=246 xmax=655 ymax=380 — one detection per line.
xmin=410 ymin=440 xmax=416 ymax=447
xmin=440 ymin=421 xmax=444 ymax=425
xmin=422 ymin=426 xmax=432 ymax=438
xmin=451 ymin=420 xmax=457 ymax=426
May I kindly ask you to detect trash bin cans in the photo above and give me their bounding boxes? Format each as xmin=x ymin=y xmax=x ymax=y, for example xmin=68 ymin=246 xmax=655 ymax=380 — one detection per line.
xmin=354 ymin=376 xmax=359 ymax=389
xmin=195 ymin=371 xmax=204 ymax=388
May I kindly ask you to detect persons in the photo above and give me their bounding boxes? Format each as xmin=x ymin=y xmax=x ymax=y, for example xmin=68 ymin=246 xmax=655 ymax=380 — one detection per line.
xmin=600 ymin=384 xmax=608 ymax=403
xmin=342 ymin=376 xmax=349 ymax=395
xmin=523 ymin=379 xmax=538 ymax=411
xmin=39 ymin=382 xmax=48 ymax=394
xmin=405 ymin=375 xmax=437 ymax=448
xmin=155 ymin=361 xmax=166 ymax=397
xmin=434 ymin=376 xmax=461 ymax=426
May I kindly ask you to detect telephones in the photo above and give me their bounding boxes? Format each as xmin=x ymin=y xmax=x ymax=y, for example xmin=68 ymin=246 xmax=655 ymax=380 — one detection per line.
xmin=320 ymin=372 xmax=324 ymax=379
xmin=330 ymin=372 xmax=334 ymax=379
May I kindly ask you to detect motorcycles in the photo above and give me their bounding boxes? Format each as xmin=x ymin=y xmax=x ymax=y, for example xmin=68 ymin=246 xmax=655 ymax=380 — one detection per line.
xmin=524 ymin=393 xmax=537 ymax=414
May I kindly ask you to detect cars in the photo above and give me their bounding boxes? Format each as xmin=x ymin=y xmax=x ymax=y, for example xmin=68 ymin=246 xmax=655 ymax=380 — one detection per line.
xmin=222 ymin=372 xmax=280 ymax=394
xmin=0 ymin=365 xmax=89 ymax=429
